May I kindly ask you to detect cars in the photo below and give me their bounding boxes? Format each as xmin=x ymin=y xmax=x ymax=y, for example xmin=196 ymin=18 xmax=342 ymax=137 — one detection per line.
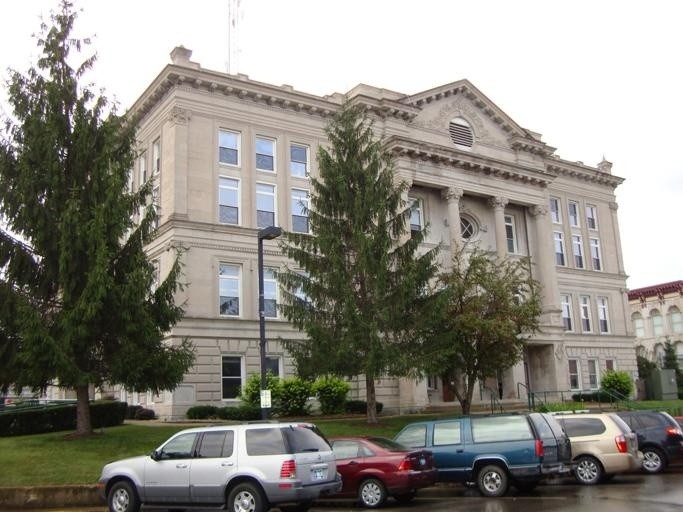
xmin=674 ymin=417 xmax=682 ymax=465
xmin=323 ymin=435 xmax=439 ymax=507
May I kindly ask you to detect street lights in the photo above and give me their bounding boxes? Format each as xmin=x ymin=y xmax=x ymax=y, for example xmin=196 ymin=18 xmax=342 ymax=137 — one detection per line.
xmin=257 ymin=226 xmax=282 ymax=421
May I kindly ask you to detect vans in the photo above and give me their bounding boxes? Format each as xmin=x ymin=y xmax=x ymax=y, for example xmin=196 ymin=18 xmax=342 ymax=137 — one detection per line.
xmin=389 ymin=412 xmax=572 ymax=498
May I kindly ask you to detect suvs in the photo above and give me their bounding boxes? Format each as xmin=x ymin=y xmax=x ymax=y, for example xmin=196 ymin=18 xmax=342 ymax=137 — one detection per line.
xmin=616 ymin=409 xmax=682 ymax=476
xmin=545 ymin=411 xmax=643 ymax=484
xmin=98 ymin=421 xmax=344 ymax=512
